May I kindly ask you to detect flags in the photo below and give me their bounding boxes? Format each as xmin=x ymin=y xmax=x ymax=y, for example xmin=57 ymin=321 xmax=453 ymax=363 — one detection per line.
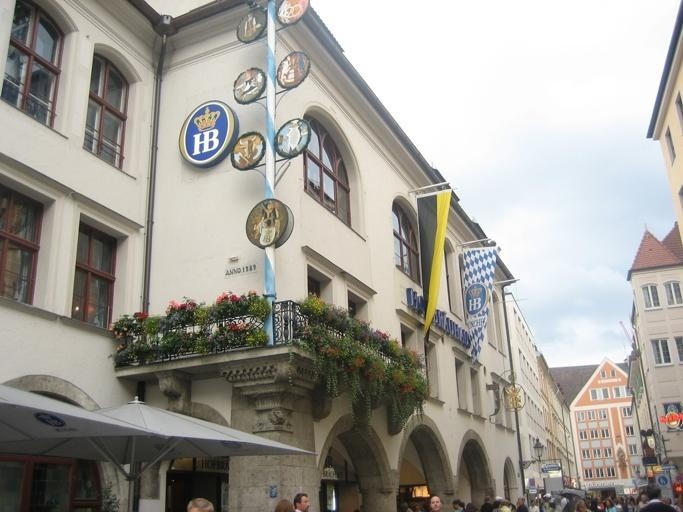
xmin=416 ymin=189 xmax=452 ymax=348
xmin=462 ymin=247 xmax=498 ymax=367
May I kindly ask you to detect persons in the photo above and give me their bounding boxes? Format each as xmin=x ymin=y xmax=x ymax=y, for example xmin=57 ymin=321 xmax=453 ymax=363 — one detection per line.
xmin=274 ymin=499 xmax=297 ymax=512
xmin=294 ymin=492 xmax=311 ymax=512
xmin=185 ymin=497 xmax=214 ymax=511
xmin=399 ymin=482 xmax=683 ymax=512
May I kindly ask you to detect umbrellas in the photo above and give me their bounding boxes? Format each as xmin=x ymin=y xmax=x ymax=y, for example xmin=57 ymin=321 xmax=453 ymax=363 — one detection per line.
xmin=0 ymin=394 xmax=320 ymax=512
xmin=0 ymin=381 xmax=170 ymax=443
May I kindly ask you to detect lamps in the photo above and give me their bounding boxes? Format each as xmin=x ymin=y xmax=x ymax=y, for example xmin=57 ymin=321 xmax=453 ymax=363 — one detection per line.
xmin=519 ymin=436 xmax=545 ymax=471
